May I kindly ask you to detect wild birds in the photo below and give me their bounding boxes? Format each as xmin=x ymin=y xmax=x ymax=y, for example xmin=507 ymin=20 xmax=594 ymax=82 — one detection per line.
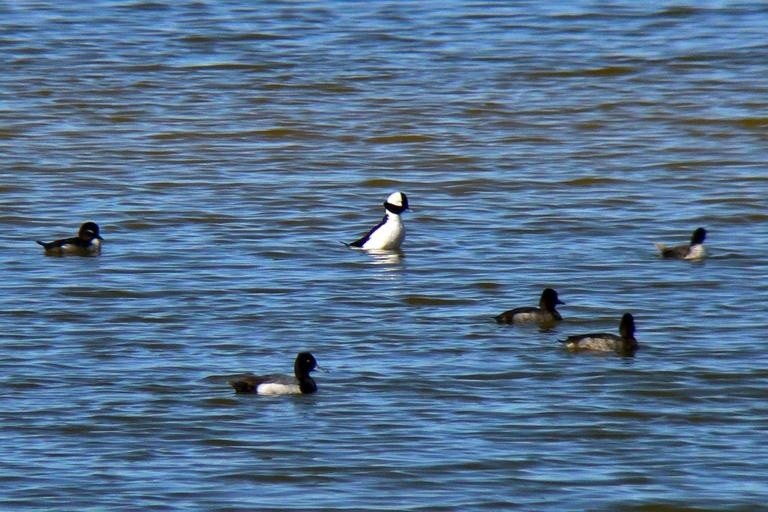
xmin=230 ymin=351 xmax=330 ymax=396
xmin=658 ymin=226 xmax=709 ymax=260
xmin=35 ymin=221 xmax=104 ymax=253
xmin=556 ymin=310 xmax=641 ymax=354
xmin=336 ymin=191 xmax=414 ymax=251
xmin=487 ymin=287 xmax=565 ymax=327
